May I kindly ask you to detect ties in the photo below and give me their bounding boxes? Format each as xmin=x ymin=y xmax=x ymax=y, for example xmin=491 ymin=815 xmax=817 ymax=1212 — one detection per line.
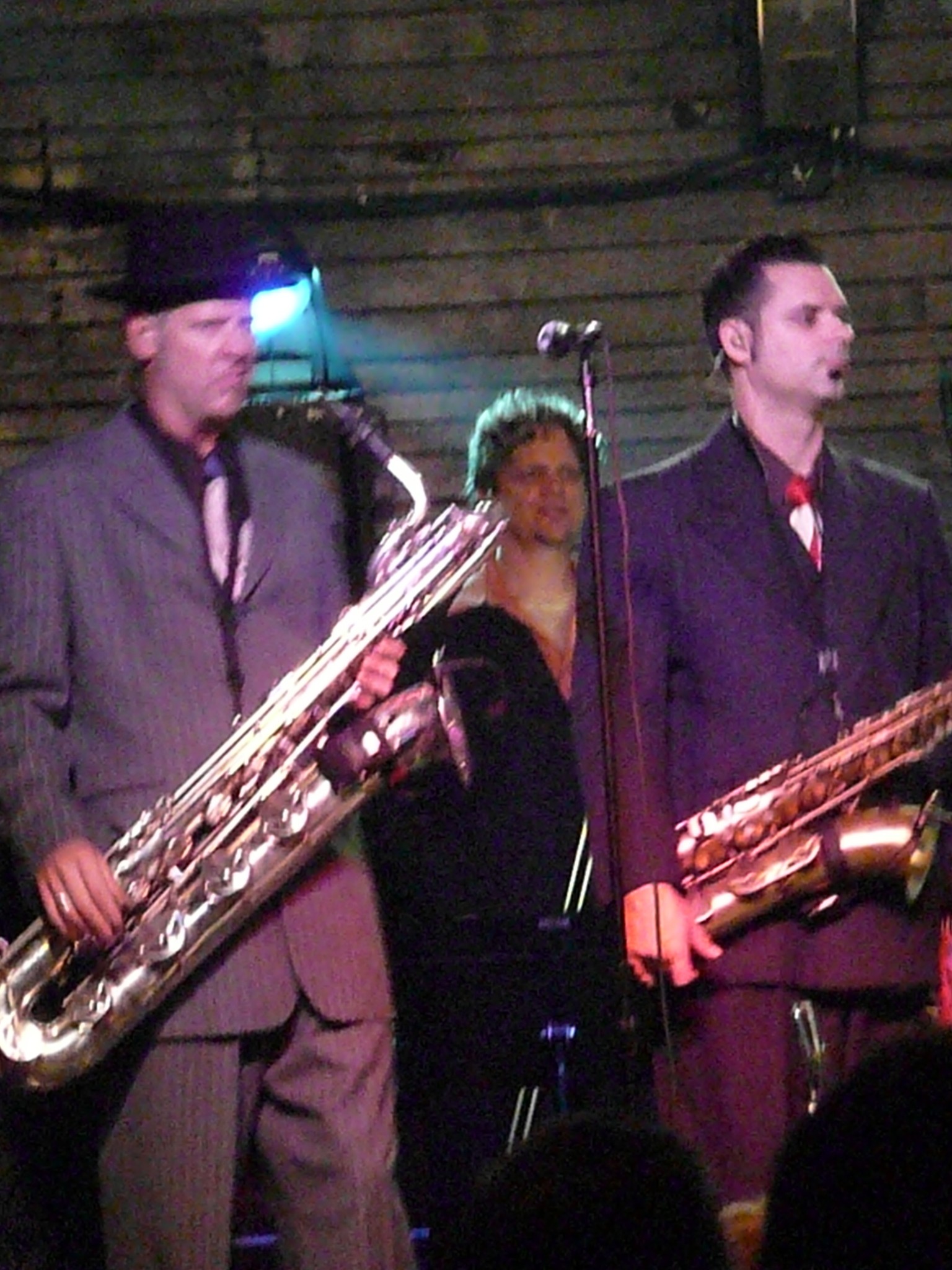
xmin=787 ymin=473 xmax=825 ymax=572
xmin=202 ymin=456 xmax=235 ymax=589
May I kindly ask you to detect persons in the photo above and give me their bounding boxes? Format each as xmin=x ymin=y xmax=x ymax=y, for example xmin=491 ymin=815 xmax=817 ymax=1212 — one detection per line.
xmin=422 ymin=1108 xmax=729 ymax=1270
xmin=569 ymin=232 xmax=952 ymax=1269
xmin=445 ymin=387 xmax=608 ymax=1120
xmin=0 ymin=202 xmax=422 ymax=1268
xmin=749 ymin=1027 xmax=952 ymax=1268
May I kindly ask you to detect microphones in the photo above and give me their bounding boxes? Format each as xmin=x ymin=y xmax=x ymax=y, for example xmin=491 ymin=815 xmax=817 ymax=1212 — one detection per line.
xmin=535 ymin=319 xmax=600 ymax=359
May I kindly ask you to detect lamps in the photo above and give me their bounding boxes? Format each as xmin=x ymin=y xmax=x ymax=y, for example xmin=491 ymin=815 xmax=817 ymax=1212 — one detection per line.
xmin=240 ymin=146 xmax=363 ymax=413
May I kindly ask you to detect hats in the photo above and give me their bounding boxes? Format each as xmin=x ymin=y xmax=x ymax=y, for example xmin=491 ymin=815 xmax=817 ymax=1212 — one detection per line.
xmin=87 ymin=211 xmax=319 ymax=317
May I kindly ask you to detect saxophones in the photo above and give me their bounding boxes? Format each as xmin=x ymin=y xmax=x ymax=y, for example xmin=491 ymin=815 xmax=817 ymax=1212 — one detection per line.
xmin=651 ymin=677 xmax=952 ymax=973
xmin=1 ymin=399 xmax=510 ymax=1093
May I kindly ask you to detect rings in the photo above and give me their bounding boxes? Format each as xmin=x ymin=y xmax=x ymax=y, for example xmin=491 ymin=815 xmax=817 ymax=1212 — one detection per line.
xmin=53 ymin=892 xmax=73 ymax=920
